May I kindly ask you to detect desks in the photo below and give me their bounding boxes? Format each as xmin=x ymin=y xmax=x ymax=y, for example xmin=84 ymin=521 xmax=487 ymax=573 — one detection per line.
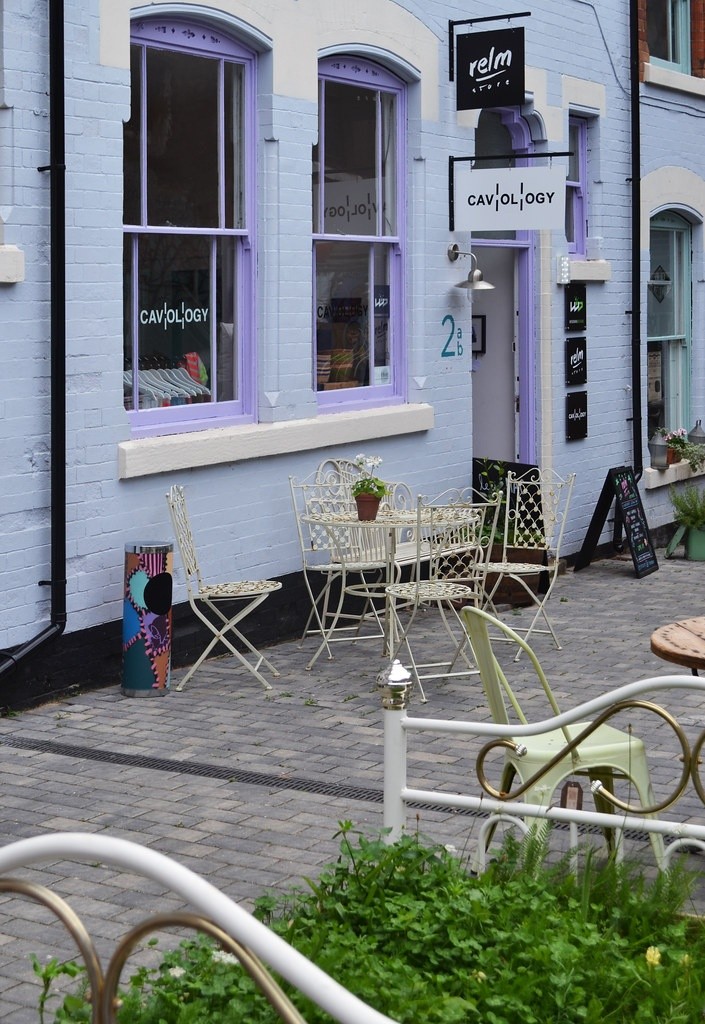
xmin=302 ymin=511 xmax=479 ymax=670
xmin=650 ymin=615 xmax=705 ymax=677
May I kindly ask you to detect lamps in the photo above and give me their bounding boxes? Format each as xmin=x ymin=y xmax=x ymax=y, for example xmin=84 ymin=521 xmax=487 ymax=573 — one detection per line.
xmin=449 ymin=242 xmax=496 ymax=291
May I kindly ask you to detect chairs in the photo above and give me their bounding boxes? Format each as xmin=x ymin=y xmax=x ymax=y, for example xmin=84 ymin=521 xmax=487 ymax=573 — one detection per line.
xmin=288 ymin=457 xmax=676 ymax=891
xmin=166 ymin=485 xmax=282 ymax=690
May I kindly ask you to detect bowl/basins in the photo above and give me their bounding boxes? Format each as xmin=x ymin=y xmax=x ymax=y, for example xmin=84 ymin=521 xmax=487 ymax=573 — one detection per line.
xmin=316 ymin=349 xmax=353 ymax=384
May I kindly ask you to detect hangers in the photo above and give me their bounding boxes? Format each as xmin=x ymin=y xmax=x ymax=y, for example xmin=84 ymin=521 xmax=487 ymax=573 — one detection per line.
xmin=124 ymin=355 xmax=214 ymax=402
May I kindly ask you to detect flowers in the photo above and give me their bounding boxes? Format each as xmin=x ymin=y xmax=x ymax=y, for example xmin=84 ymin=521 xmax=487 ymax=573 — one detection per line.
xmin=350 ymin=454 xmax=395 ymax=499
xmin=472 ymin=458 xmax=567 ymax=545
xmin=662 ymin=429 xmax=705 ymax=475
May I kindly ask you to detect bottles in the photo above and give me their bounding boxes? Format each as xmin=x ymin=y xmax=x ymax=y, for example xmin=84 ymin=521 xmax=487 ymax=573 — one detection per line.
xmin=688 ymin=419 xmax=705 ymax=443
xmin=650 ymin=427 xmax=669 ymax=469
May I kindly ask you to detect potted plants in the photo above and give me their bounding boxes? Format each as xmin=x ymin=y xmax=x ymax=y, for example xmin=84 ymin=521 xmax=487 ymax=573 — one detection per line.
xmin=664 ymin=485 xmax=705 ymax=561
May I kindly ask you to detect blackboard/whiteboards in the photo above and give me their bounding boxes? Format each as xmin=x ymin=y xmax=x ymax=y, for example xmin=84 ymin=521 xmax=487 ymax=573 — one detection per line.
xmin=574 ymin=465 xmax=660 ymax=579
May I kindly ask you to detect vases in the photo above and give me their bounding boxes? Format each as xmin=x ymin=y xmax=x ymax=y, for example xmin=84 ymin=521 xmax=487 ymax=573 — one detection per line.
xmin=317 ymin=354 xmax=331 ymax=384
xmin=480 ymin=540 xmax=547 ymax=606
xmin=668 ymin=447 xmax=680 ymax=464
xmin=354 ymin=493 xmax=381 ymax=520
xmin=323 ymin=349 xmax=355 ymax=382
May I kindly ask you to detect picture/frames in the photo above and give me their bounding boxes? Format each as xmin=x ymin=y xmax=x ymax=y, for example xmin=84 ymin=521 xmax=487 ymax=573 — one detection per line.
xmin=471 ymin=315 xmax=487 ymax=355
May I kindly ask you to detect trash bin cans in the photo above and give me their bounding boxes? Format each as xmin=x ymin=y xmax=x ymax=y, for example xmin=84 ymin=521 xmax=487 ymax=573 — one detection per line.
xmin=120 ymin=541 xmax=172 ymax=697
